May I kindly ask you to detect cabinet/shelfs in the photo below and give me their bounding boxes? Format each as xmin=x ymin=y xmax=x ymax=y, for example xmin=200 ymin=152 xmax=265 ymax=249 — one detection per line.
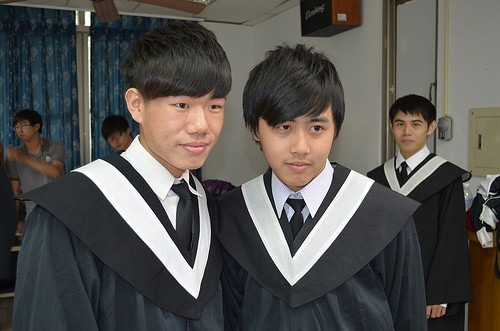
xmin=300 ymin=0 xmax=362 ymax=38
xmin=467 ymin=108 xmax=500 ymax=178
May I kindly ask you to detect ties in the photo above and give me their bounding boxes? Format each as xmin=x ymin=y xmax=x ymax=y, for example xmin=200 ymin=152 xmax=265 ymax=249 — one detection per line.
xmin=286 ymin=199 xmax=306 ymax=241
xmin=400 ymin=162 xmax=408 ymax=186
xmin=171 ymin=181 xmax=193 ymax=253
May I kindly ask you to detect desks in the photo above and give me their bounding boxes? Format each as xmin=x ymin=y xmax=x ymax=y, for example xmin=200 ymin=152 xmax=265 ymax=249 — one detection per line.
xmin=465 ymin=226 xmax=500 ymax=331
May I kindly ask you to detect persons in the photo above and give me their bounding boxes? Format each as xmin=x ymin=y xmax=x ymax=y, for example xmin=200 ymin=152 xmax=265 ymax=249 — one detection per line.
xmin=5 ymin=109 xmax=65 ymax=232
xmin=0 ymin=153 xmax=18 ymax=280
xmin=102 ymin=114 xmax=134 ymax=154
xmin=366 ymin=95 xmax=472 ymax=331
xmin=213 ymin=40 xmax=428 ymax=331
xmin=13 ymin=20 xmax=231 ymax=331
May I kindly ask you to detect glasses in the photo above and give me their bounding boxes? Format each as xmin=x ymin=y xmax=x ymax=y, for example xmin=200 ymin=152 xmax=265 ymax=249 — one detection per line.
xmin=108 ymin=132 xmax=125 ymax=142
xmin=13 ymin=123 xmax=31 ymax=130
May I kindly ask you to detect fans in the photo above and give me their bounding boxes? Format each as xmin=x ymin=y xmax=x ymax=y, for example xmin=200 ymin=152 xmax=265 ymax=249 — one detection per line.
xmin=0 ymin=0 xmax=205 ymax=24
